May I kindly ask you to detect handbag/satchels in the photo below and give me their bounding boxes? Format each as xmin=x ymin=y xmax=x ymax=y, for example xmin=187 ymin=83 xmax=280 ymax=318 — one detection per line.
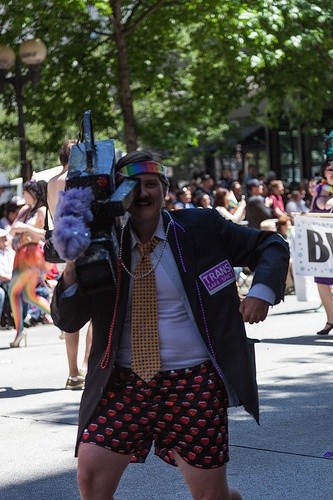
xmin=42 ymin=182 xmax=66 ymax=263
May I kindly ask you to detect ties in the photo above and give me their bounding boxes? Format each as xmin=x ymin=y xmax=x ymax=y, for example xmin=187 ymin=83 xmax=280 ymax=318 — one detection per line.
xmin=130 ymin=236 xmax=161 ymax=383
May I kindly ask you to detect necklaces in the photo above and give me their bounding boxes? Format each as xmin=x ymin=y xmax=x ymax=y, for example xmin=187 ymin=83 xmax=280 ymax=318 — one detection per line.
xmin=100 ymin=211 xmax=224 ymax=378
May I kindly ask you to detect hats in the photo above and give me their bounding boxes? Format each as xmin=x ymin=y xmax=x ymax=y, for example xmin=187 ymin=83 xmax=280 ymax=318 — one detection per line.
xmin=245 ymin=179 xmax=262 ymax=186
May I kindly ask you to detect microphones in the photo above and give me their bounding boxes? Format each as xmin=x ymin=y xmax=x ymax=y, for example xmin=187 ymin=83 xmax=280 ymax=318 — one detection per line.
xmin=48 ymin=186 xmax=96 ymax=262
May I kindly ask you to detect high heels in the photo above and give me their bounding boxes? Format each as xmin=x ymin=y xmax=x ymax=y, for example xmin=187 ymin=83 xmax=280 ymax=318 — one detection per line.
xmin=59 ymin=330 xmax=65 ymax=339
xmin=316 ymin=322 xmax=333 ymax=335
xmin=10 ymin=329 xmax=28 ymax=347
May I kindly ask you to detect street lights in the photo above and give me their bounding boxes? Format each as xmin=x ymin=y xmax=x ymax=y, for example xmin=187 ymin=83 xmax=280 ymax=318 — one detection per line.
xmin=0 ymin=39 xmax=48 ymax=180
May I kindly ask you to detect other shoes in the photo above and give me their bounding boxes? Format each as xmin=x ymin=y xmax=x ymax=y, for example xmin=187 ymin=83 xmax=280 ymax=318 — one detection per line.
xmin=65 ymin=376 xmax=85 ymax=389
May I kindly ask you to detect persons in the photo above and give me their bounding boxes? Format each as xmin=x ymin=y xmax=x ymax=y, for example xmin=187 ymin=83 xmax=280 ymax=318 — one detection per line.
xmin=309 ymin=158 xmax=333 ymax=335
xmin=165 ymin=165 xmax=322 ymax=301
xmin=0 ymin=196 xmax=66 ymax=328
xmin=50 ymin=151 xmax=290 ymax=500
xmin=10 ymin=180 xmax=65 ymax=347
xmin=47 ymin=140 xmax=92 ymax=389
